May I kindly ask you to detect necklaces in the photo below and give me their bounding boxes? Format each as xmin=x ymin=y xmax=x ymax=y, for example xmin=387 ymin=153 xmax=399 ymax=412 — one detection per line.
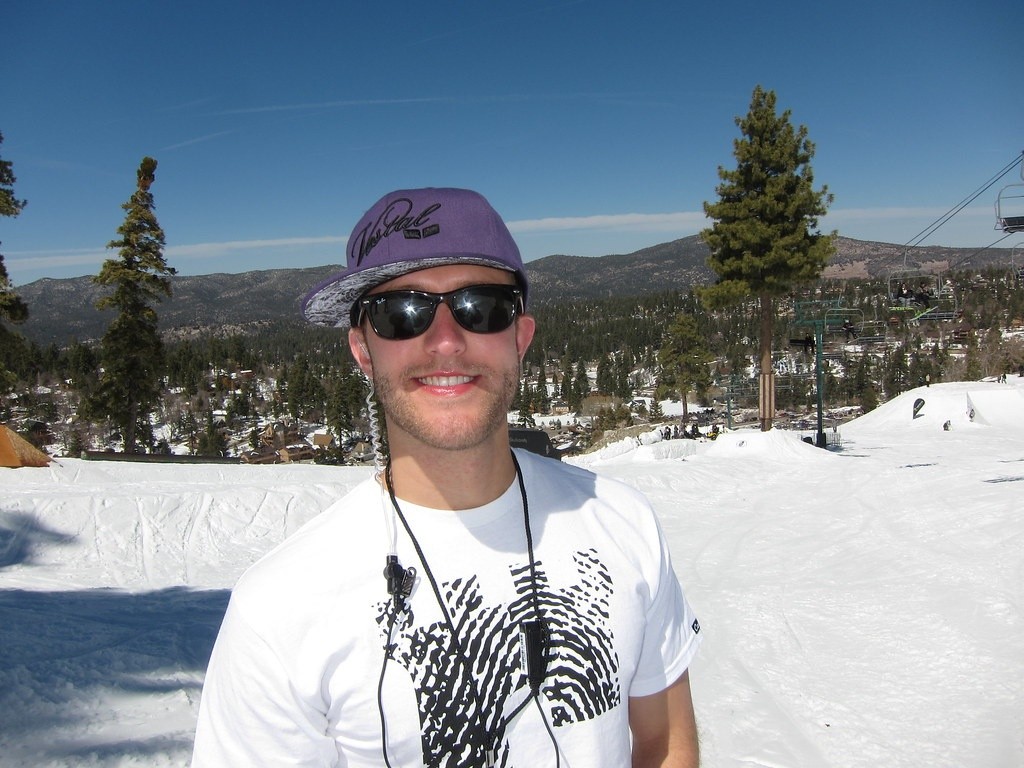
xmin=385 ymin=447 xmax=550 ymax=748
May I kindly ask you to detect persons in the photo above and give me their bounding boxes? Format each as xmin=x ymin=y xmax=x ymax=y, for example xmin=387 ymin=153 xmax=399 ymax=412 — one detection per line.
xmin=660 ymin=423 xmax=719 ymax=440
xmin=192 ymin=189 xmax=702 ymax=768
xmin=893 ymin=281 xmax=953 ymax=314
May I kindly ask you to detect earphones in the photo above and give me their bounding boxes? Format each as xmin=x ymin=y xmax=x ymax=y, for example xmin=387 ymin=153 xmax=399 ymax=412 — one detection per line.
xmin=358 ymin=340 xmax=371 ymax=360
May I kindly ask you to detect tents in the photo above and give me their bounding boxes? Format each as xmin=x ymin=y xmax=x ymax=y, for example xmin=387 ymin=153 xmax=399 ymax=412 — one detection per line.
xmin=0 ymin=424 xmax=62 ymax=468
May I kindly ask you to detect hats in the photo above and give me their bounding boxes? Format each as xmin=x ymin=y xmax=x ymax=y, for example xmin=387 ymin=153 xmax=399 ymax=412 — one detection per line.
xmin=300 ymin=187 xmax=529 ymax=328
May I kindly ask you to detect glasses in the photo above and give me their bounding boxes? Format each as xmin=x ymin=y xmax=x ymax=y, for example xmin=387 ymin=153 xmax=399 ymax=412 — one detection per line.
xmin=348 ymin=284 xmax=529 ymax=343
